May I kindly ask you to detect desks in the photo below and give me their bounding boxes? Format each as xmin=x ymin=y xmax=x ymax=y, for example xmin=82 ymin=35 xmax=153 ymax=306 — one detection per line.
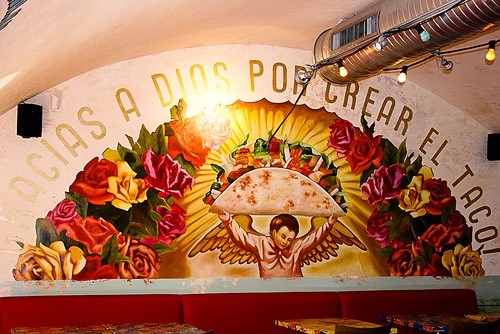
xmin=11 ymin=326 xmax=112 ymax=334
xmin=463 ymin=312 xmax=500 ymax=325
xmin=276 ymin=317 xmax=386 ymax=334
xmin=383 ymin=314 xmax=485 ymax=334
xmin=102 ymin=322 xmax=209 ymax=334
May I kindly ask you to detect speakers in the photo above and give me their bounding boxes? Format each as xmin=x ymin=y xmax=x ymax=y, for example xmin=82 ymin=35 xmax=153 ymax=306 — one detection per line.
xmin=487 ymin=133 xmax=500 ymax=160
xmin=16 ymin=104 xmax=43 ymax=138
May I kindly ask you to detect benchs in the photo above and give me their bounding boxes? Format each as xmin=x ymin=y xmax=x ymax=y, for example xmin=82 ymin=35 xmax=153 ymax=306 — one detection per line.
xmin=0 ymin=288 xmax=477 ymax=334
